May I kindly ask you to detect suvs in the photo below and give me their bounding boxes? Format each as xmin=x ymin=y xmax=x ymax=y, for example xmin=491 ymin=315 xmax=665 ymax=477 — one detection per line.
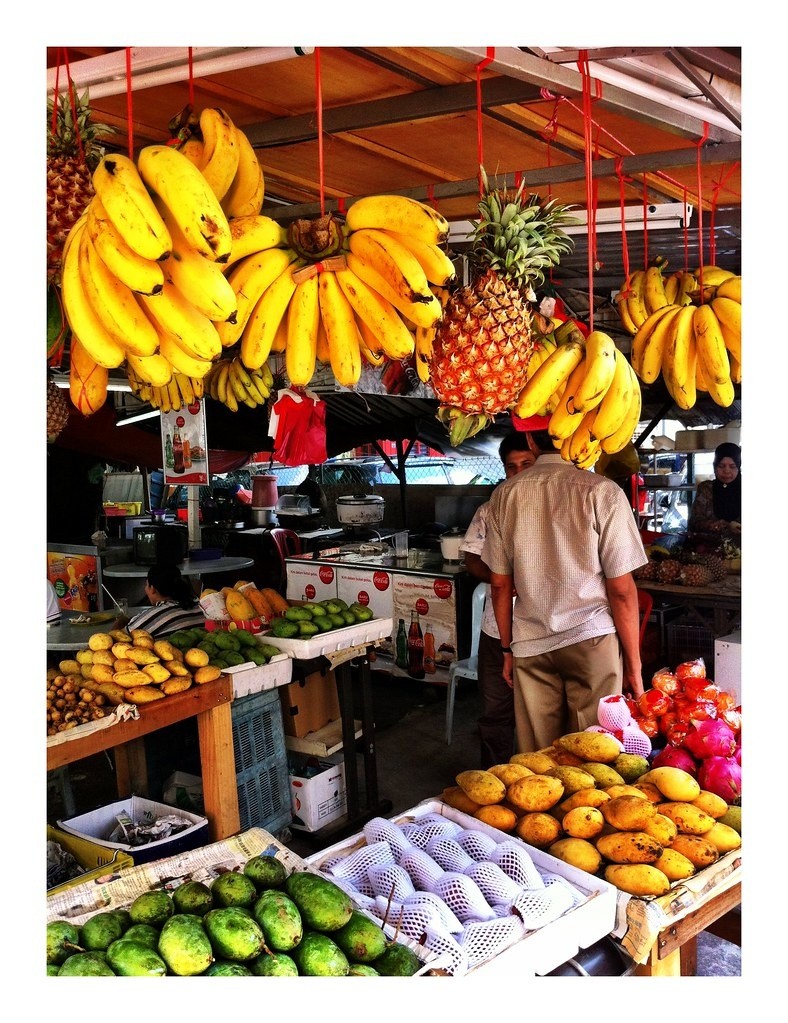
xmin=224 ymin=453 xmax=491 ymax=486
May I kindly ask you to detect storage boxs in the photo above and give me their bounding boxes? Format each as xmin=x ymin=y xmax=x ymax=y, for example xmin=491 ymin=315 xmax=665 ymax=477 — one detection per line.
xmin=704 ymin=428 xmax=740 ymax=449
xmin=55 ymin=793 xmax=209 ymax=865
xmin=47 ymin=824 xmax=134 ymax=897
xmin=675 ymin=430 xmax=705 ymax=449
xmin=288 ymin=753 xmax=345 ymax=834
xmin=299 ymin=798 xmax=619 ymax=976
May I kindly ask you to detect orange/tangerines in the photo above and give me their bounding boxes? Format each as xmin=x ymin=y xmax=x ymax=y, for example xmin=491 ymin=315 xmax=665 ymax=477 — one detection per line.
xmin=625 ymin=661 xmax=741 ymax=744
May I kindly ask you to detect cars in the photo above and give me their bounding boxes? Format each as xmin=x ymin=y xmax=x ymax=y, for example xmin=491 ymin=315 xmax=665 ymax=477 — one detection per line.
xmin=646 ymin=453 xmax=716 ymax=534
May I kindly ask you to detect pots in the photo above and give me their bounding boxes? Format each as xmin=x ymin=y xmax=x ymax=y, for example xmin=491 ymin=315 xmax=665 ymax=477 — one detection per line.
xmin=145 ymin=510 xmax=166 ymax=523
xmin=335 ymin=494 xmax=386 ymax=523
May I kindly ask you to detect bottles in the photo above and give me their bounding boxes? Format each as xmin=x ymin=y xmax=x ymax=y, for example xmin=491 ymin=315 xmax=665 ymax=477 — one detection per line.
xmin=166 ymin=426 xmax=192 ymax=473
xmin=395 ymin=610 xmax=436 ymax=680
xmin=64 ymin=563 xmax=97 ymax=612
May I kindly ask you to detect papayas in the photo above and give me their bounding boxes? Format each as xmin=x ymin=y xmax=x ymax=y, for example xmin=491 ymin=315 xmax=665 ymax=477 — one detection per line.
xmin=169 ymin=627 xmax=280 ymax=669
xmin=47 ymin=854 xmax=419 ymax=976
xmin=270 ymin=598 xmax=373 ymax=639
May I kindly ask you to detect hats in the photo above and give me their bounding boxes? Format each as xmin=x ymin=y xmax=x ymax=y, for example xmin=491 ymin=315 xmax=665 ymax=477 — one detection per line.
xmin=714 ymin=442 xmax=741 ymax=470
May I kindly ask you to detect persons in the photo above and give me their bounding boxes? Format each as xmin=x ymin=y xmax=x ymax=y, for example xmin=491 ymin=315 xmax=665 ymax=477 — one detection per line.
xmin=482 ymin=429 xmax=648 ymax=752
xmin=687 ymin=442 xmax=741 ymax=536
xmin=458 ymin=432 xmax=536 ymax=773
xmin=98 ymin=560 xmax=207 ymax=798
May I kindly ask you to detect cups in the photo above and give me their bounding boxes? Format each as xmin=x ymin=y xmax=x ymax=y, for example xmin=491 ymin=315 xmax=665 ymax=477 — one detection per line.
xmin=113 ymin=598 xmax=128 ymax=621
xmin=392 ymin=533 xmax=408 ymax=558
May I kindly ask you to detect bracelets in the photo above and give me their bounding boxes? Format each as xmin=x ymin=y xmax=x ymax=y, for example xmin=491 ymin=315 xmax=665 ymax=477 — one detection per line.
xmin=501 ymin=647 xmax=511 ymax=652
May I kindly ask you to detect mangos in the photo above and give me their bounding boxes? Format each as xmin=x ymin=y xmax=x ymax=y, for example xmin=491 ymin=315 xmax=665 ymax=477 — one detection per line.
xmin=60 ymin=631 xmax=222 ymax=704
xmin=200 ymin=580 xmax=288 ymax=622
xmin=443 ymin=732 xmax=741 ymax=892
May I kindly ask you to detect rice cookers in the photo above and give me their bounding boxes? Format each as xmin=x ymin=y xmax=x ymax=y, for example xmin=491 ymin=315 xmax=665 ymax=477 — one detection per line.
xmin=436 ymin=527 xmax=468 ymax=562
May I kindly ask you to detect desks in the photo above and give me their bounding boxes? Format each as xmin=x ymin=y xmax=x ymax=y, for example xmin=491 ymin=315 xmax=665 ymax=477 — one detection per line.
xmin=47 ymin=605 xmax=154 ymax=651
xmin=103 ymin=556 xmax=254 ymax=591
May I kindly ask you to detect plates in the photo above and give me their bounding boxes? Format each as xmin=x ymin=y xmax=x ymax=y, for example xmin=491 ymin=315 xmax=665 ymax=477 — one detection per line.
xmin=68 ymin=613 xmax=114 ymax=626
xmin=191 ymin=458 xmax=205 ymax=461
xmin=435 ymin=664 xmax=449 ymax=669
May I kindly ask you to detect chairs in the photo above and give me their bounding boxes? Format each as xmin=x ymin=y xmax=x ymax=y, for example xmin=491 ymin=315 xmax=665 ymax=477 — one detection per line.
xmin=623 ymin=588 xmax=653 ymax=692
xmin=445 ymin=582 xmax=488 ymax=748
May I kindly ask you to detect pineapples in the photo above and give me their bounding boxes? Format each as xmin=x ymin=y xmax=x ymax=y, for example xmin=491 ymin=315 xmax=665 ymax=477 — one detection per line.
xmin=429 ymin=165 xmax=583 ymax=446
xmin=47 ymin=383 xmax=69 ymax=441
xmin=267 ymin=373 xmax=284 ymax=420
xmin=46 ymin=75 xmax=118 ymax=288
xmin=633 ymin=545 xmax=727 ymax=586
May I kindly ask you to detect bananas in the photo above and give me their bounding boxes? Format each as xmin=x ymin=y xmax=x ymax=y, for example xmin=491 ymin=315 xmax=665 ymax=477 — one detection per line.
xmin=60 ymin=107 xmax=456 ymax=411
xmin=622 ymin=265 xmax=741 ymax=409
xmin=515 ymin=318 xmax=640 ymax=466
